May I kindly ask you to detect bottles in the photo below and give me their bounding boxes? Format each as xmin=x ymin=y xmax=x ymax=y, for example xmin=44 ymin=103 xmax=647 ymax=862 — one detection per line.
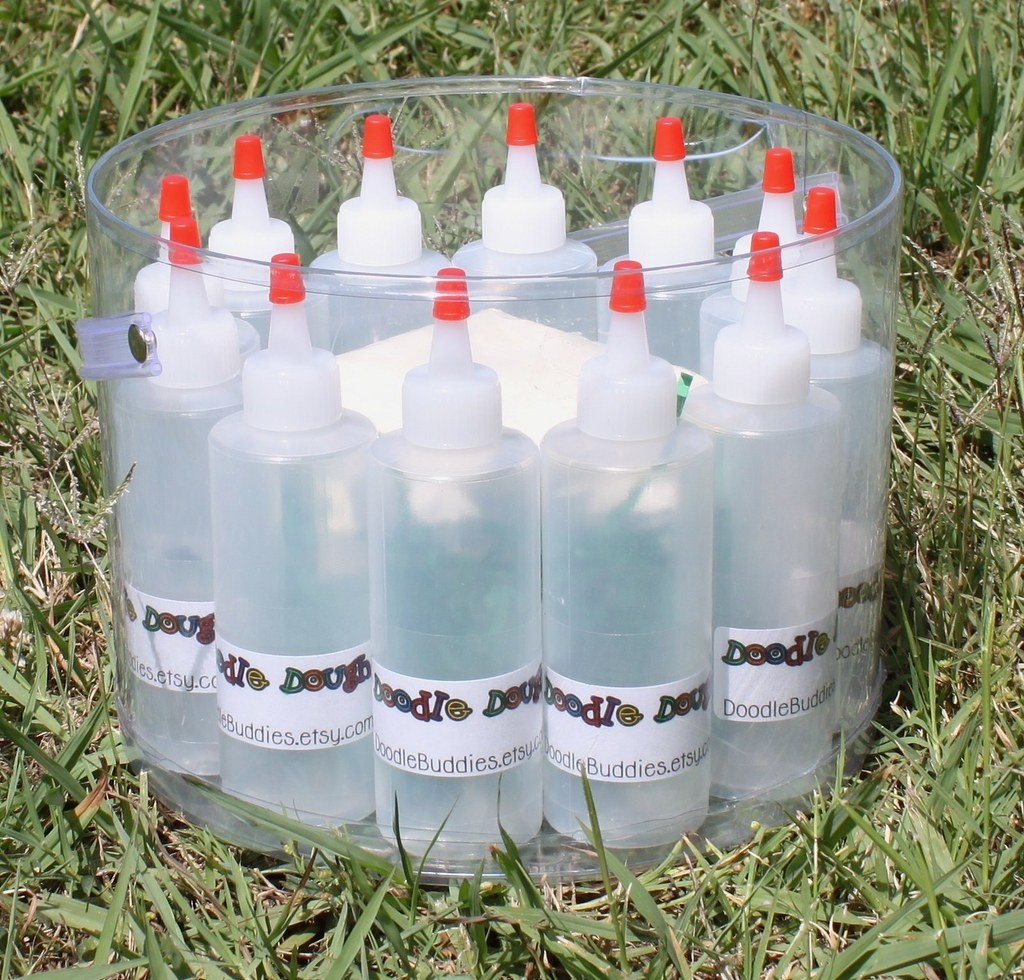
xmin=111 ymin=102 xmax=898 ymax=861
